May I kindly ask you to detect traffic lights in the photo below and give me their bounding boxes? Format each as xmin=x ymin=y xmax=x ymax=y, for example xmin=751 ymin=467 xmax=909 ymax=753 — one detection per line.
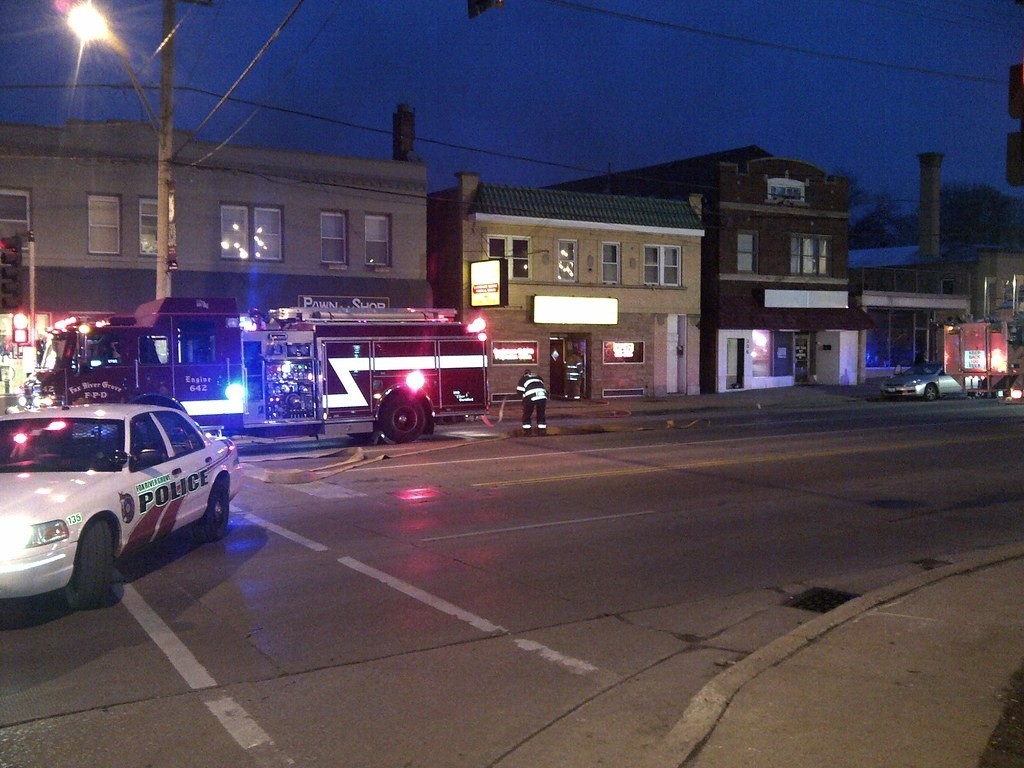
xmin=0 ymin=231 xmax=25 ymax=310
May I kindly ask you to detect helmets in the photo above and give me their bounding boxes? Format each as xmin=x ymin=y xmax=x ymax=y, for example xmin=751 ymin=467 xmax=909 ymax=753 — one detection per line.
xmin=522 ymin=369 xmax=531 ymax=377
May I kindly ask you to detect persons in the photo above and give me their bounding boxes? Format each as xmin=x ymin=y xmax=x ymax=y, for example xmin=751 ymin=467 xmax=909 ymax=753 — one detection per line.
xmin=563 ymin=348 xmax=584 ymax=400
xmin=517 ymin=369 xmax=549 ymax=436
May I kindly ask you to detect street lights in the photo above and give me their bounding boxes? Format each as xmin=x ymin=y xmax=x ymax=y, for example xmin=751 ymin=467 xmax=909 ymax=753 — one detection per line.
xmin=69 ymin=3 xmax=178 ymax=298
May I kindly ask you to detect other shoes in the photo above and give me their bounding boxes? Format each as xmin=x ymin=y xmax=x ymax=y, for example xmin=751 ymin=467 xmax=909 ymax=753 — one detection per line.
xmin=572 ymin=399 xmax=581 ymax=402
xmin=522 ymin=429 xmax=531 ymax=436
xmin=561 ymin=398 xmax=567 ymax=402
xmin=537 ymin=429 xmax=546 ymax=436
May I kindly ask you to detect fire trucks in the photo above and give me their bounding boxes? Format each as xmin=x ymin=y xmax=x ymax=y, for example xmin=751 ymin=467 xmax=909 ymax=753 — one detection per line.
xmin=28 ymin=294 xmax=488 ymax=447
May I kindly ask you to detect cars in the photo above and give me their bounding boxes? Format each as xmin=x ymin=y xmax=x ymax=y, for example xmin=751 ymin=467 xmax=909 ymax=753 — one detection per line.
xmin=881 ymin=362 xmax=984 ymax=401
xmin=1 ymin=406 xmax=247 ymax=609
